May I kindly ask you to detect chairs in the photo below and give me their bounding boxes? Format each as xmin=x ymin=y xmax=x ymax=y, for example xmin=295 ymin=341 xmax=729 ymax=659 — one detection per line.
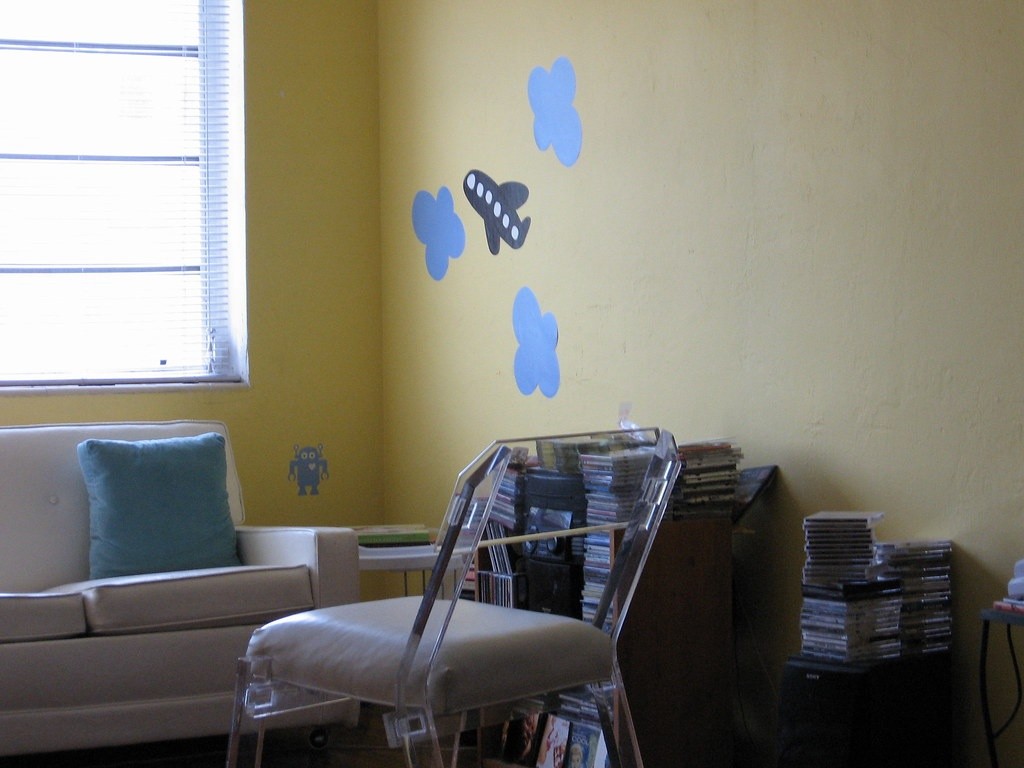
xmin=227 ymin=426 xmax=685 ymax=768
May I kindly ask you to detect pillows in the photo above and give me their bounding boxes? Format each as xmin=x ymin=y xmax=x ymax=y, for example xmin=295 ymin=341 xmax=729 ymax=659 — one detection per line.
xmin=77 ymin=432 xmax=242 ymax=580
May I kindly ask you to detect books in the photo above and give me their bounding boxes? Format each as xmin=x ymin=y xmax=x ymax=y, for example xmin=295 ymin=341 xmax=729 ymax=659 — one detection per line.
xmin=500 ymin=681 xmax=616 ymax=768
xmin=350 ymin=523 xmax=429 ymax=544
xmin=799 ymin=510 xmax=954 ymax=664
xmin=456 ymin=435 xmax=776 ymax=635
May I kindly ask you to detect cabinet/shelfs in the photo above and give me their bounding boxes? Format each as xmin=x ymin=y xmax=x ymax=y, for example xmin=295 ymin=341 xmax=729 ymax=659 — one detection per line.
xmin=474 ymin=516 xmax=733 ymax=768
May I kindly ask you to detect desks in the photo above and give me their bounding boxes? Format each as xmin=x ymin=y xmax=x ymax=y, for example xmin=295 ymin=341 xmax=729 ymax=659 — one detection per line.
xmin=980 ymin=608 xmax=1024 ymax=768
xmin=360 ymin=554 xmax=464 ymax=600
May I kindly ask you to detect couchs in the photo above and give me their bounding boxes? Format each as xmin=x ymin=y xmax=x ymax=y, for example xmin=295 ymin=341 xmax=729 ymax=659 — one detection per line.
xmin=0 ymin=421 xmax=362 ymax=768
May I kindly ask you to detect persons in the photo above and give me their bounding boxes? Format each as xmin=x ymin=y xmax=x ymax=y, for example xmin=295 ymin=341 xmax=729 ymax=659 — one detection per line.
xmin=504 ymin=712 xmax=540 ymax=758
xmin=570 ymin=744 xmax=582 ymax=768
xmin=538 ymin=714 xmax=555 ymax=764
